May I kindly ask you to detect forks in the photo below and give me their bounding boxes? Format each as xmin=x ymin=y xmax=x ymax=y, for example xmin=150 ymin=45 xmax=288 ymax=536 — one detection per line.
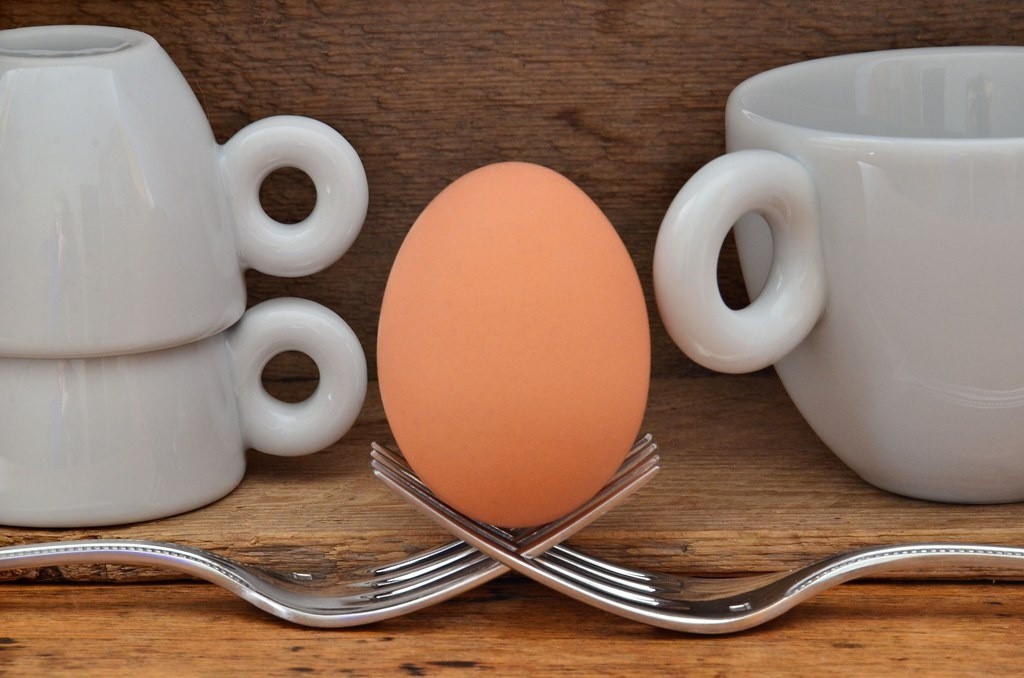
xmin=371 ymin=442 xmax=1024 ymax=633
xmin=0 ymin=425 xmax=661 ymax=628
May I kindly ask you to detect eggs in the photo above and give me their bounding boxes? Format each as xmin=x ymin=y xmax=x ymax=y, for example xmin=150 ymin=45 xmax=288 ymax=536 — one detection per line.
xmin=377 ymin=162 xmax=650 ymax=529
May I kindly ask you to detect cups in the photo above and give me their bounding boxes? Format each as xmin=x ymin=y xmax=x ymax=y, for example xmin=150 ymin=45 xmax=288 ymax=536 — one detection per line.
xmin=652 ymin=46 xmax=1024 ymax=503
xmin=0 ymin=297 xmax=368 ymax=527
xmin=0 ymin=25 xmax=371 ymax=359
